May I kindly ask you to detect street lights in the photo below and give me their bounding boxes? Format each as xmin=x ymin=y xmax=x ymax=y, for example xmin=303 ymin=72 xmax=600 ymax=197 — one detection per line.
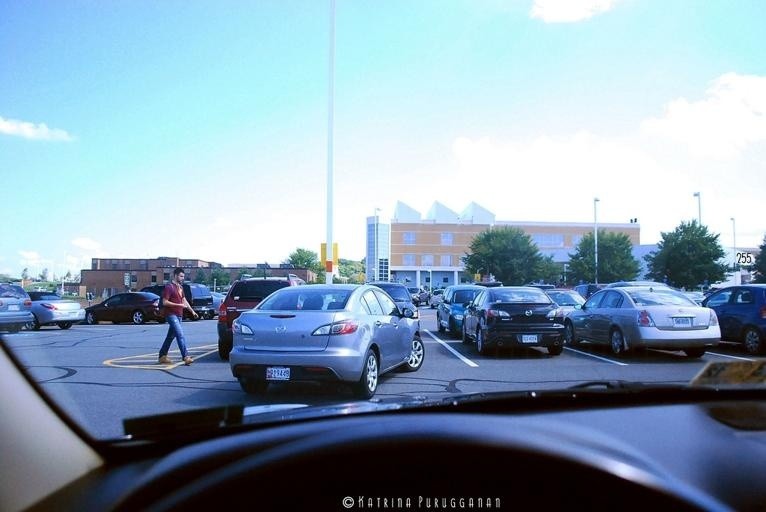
xmin=693 ymin=191 xmax=702 ymax=226
xmin=561 ymin=264 xmax=570 ymax=287
xmin=593 ymin=197 xmax=598 ymax=281
xmin=730 ymin=217 xmax=737 ymax=272
xmin=426 ymin=268 xmax=432 ymax=293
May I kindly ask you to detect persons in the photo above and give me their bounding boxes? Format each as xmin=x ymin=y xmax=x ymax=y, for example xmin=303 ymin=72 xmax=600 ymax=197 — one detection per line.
xmin=158 ymin=267 xmax=199 ymax=366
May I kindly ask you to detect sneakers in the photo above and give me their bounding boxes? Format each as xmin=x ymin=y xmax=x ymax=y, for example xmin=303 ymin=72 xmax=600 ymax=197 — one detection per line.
xmin=159 ymin=357 xmax=172 ymax=363
xmin=184 ymin=356 xmax=193 ymax=365
